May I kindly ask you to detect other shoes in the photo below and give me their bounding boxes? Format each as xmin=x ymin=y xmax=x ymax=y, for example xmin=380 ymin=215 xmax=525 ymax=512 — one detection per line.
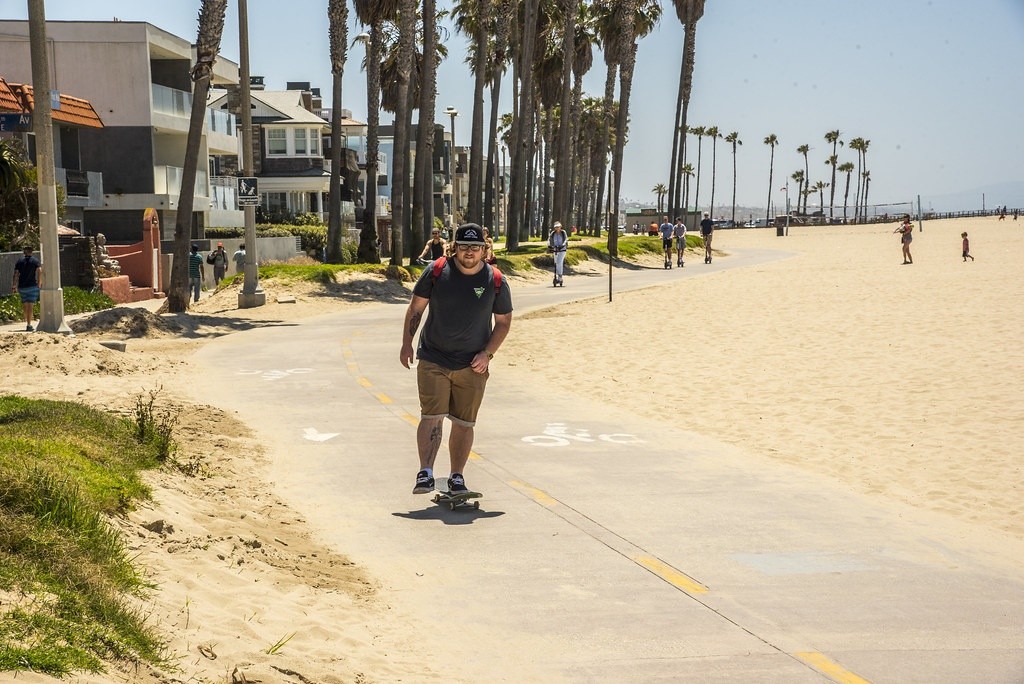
xmin=558 ymin=276 xmax=562 ymax=282
xmin=26 ymin=326 xmax=33 ymax=330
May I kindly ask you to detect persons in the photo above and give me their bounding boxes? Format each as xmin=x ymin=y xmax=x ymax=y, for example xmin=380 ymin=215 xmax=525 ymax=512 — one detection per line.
xmin=482 ymin=227 xmax=497 ymax=268
xmin=997 ymin=205 xmax=1018 ymax=221
xmin=549 ymin=222 xmax=568 ymax=280
xmin=12 ymin=245 xmax=42 ymax=331
xmin=894 ymin=214 xmax=914 ymax=264
xmin=660 ymin=215 xmax=686 ymax=262
xmin=700 ymin=212 xmax=714 ymax=259
xmin=209 ymin=242 xmax=229 ymax=285
xmin=400 ymin=223 xmax=513 ymax=495
xmin=232 ymin=243 xmax=245 ymax=274
xmin=189 ymin=244 xmax=205 ymax=302
xmin=633 ymin=221 xmax=658 ymax=236
xmin=961 ymin=232 xmax=974 ymax=262
xmin=417 ymin=226 xmax=455 ymax=260
xmin=97 ymin=232 xmax=119 ymax=269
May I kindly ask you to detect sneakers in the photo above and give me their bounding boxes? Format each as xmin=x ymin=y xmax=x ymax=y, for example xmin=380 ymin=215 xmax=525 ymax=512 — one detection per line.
xmin=412 ymin=473 xmax=433 ymax=493
xmin=447 ymin=472 xmax=468 ymax=494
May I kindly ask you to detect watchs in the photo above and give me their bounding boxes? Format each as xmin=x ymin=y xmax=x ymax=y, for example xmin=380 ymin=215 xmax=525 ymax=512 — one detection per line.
xmin=484 ymin=349 xmax=493 ymax=361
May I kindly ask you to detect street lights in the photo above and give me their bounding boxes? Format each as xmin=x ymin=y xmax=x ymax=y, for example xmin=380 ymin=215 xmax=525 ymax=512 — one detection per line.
xmin=493 ymin=138 xmax=507 ymax=243
xmin=443 ymin=105 xmax=460 ymax=241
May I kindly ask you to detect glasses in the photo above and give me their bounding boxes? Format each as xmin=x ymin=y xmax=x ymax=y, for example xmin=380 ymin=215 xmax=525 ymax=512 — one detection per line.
xmin=458 ymin=244 xmax=482 ymax=252
xmin=433 ymin=233 xmax=438 ymax=235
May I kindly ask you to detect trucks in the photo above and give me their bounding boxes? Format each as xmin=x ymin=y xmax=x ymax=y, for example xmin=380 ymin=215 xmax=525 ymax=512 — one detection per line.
xmin=744 ymin=218 xmax=775 ymax=228
xmin=711 ymin=219 xmax=733 ymax=228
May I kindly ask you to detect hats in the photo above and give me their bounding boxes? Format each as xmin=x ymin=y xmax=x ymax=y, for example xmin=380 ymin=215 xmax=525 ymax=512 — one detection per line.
xmin=217 ymin=243 xmax=223 ymax=246
xmin=454 ymin=224 xmax=486 ymax=248
xmin=554 ymin=224 xmax=562 ymax=227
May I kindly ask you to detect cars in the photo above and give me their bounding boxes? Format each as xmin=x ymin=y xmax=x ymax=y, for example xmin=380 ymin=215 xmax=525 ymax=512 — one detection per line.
xmin=601 ymin=224 xmax=628 ymax=233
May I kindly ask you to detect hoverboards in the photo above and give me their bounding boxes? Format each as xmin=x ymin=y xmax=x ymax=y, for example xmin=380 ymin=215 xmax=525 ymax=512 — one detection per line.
xmin=673 ymin=237 xmax=685 ymax=267
xmin=550 ymin=246 xmax=567 ymax=287
xmin=701 ymin=234 xmax=712 ymax=264
xmin=662 ymin=237 xmax=672 ymax=270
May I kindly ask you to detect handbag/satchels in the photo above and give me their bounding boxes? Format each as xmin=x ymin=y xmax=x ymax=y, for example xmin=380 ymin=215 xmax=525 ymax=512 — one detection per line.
xmin=207 ymin=251 xmax=216 ymax=265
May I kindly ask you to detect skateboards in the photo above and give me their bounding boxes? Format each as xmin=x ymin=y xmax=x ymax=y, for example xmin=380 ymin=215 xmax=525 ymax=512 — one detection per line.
xmin=431 ymin=476 xmax=483 ymax=511
